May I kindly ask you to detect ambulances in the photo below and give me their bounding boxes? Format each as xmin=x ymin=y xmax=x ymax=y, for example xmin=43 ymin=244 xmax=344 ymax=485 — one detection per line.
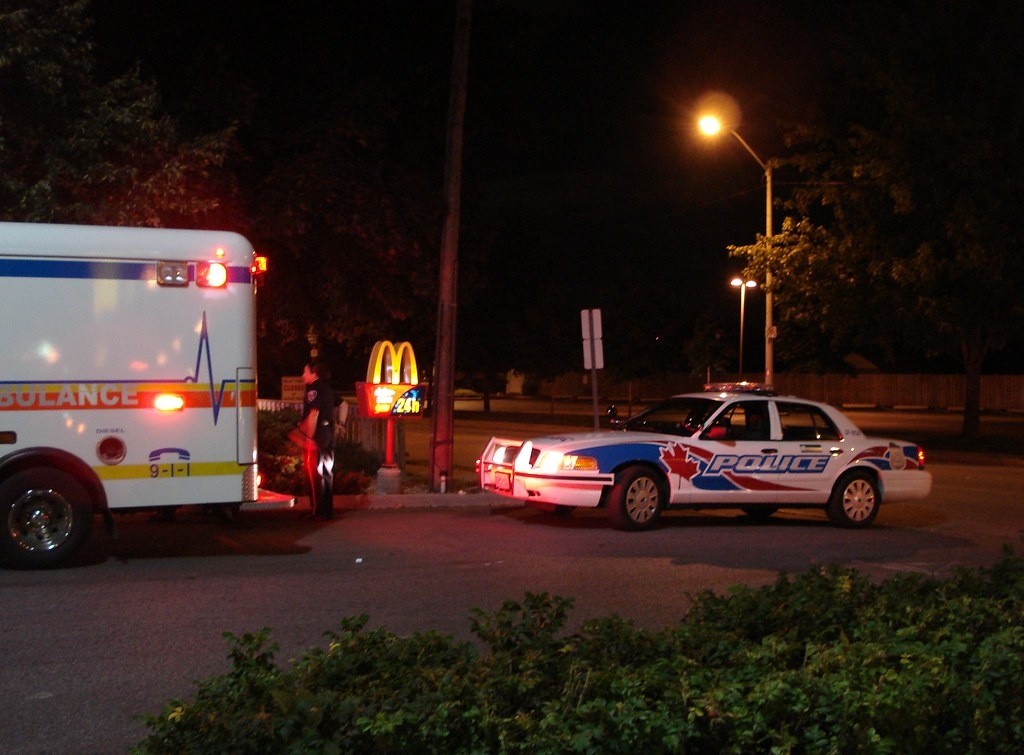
xmin=0 ymin=219 xmax=299 ymax=569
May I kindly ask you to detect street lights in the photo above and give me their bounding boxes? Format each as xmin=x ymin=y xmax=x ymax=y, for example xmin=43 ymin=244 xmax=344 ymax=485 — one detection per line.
xmin=696 ymin=110 xmax=774 ymax=393
xmin=729 ymin=276 xmax=758 ymax=382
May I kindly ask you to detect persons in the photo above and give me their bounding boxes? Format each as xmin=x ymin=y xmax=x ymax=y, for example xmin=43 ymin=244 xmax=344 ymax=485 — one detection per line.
xmin=288 ymin=361 xmax=349 ymax=522
xmin=707 ymin=409 xmax=770 ymax=440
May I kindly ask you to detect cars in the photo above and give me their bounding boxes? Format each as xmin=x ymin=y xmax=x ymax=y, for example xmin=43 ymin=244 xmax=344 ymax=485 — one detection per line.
xmin=474 ymin=380 xmax=934 ymax=534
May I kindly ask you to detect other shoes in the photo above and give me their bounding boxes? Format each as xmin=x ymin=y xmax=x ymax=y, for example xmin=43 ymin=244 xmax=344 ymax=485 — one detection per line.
xmin=304 ymin=513 xmax=332 ymax=521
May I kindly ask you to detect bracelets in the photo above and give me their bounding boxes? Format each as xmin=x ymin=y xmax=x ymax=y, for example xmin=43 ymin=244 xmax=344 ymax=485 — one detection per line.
xmin=337 ymin=421 xmax=344 ymax=427
